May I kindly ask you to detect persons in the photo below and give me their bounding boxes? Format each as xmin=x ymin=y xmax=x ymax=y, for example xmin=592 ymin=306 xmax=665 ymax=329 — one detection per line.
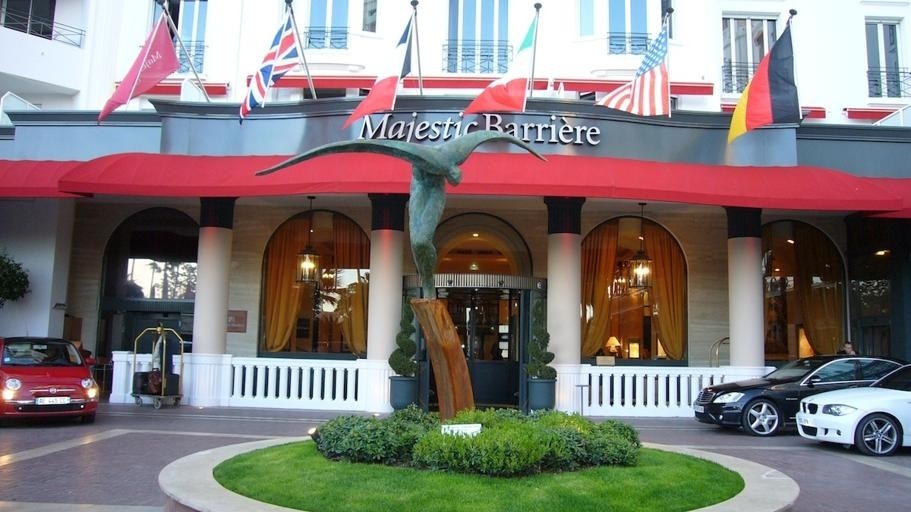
xmin=837 ymin=341 xmax=856 ymax=355
xmin=489 ymin=336 xmax=502 ymax=360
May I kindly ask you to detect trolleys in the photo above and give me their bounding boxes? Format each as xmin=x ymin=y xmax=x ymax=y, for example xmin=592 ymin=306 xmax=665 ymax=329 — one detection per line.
xmin=130 ymin=324 xmax=185 ymax=410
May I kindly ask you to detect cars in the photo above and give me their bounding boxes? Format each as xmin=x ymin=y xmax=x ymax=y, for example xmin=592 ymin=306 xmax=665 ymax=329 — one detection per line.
xmin=0 ymin=337 xmax=100 ymax=425
xmin=693 ymin=355 xmax=911 ymax=458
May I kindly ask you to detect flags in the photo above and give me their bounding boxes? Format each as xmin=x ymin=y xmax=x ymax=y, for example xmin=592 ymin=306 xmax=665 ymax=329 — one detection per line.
xmin=728 ymin=17 xmax=803 ymax=144
xmin=594 ymin=13 xmax=671 ymax=117
xmin=342 ymin=9 xmax=416 ymax=130
xmin=239 ymin=5 xmax=303 ymax=127
xmin=463 ymin=12 xmax=537 ymax=115
xmin=98 ymin=10 xmax=182 ymax=125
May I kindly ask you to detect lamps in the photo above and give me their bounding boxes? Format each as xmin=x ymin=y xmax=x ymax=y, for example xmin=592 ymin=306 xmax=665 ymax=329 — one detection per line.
xmin=292 ymin=195 xmax=322 ymax=284
xmin=625 ymin=202 xmax=656 ymax=290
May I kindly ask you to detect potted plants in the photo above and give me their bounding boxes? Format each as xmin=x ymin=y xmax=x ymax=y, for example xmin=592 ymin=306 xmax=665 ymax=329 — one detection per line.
xmin=387 ymin=297 xmax=420 ymax=412
xmin=524 ymin=296 xmax=557 ymax=412
